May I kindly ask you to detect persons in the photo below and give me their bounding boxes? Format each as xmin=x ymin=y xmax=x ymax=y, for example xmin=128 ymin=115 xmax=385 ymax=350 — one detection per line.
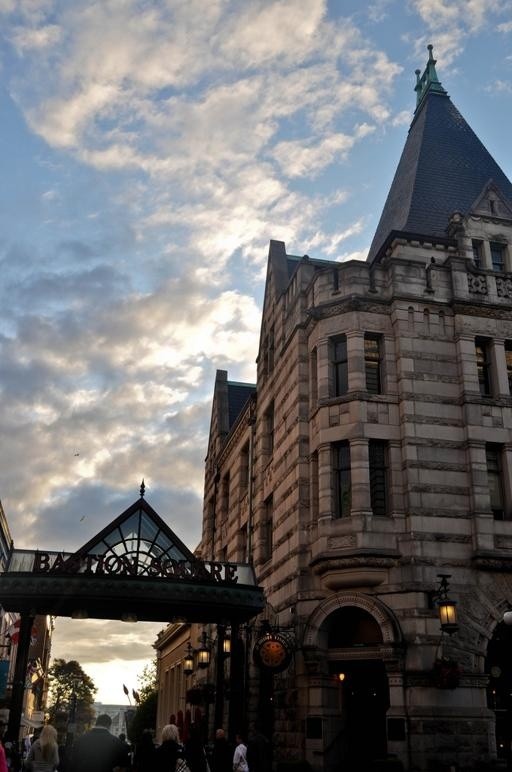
xmin=232 ymin=732 xmax=251 ymax=771
xmin=1 ymin=711 xmax=212 ymax=772
xmin=212 ymin=728 xmax=232 ymax=771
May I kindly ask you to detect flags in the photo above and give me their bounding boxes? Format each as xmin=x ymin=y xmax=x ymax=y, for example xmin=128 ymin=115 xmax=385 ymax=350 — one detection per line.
xmin=27 ymin=657 xmax=46 ymax=687
xmin=8 ymin=618 xmax=39 ymax=646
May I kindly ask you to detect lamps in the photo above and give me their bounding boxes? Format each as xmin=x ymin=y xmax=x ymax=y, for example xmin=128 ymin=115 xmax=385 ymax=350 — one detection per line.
xmin=434 ymin=569 xmax=461 ymax=638
xmin=219 ymin=624 xmax=232 ymax=655
xmin=197 ymin=632 xmax=211 ymax=669
xmin=183 ymin=642 xmax=194 ymax=676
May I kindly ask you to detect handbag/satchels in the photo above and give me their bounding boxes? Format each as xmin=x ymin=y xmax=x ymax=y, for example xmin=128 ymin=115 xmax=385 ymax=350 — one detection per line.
xmin=175 ymin=758 xmax=190 ymax=772
